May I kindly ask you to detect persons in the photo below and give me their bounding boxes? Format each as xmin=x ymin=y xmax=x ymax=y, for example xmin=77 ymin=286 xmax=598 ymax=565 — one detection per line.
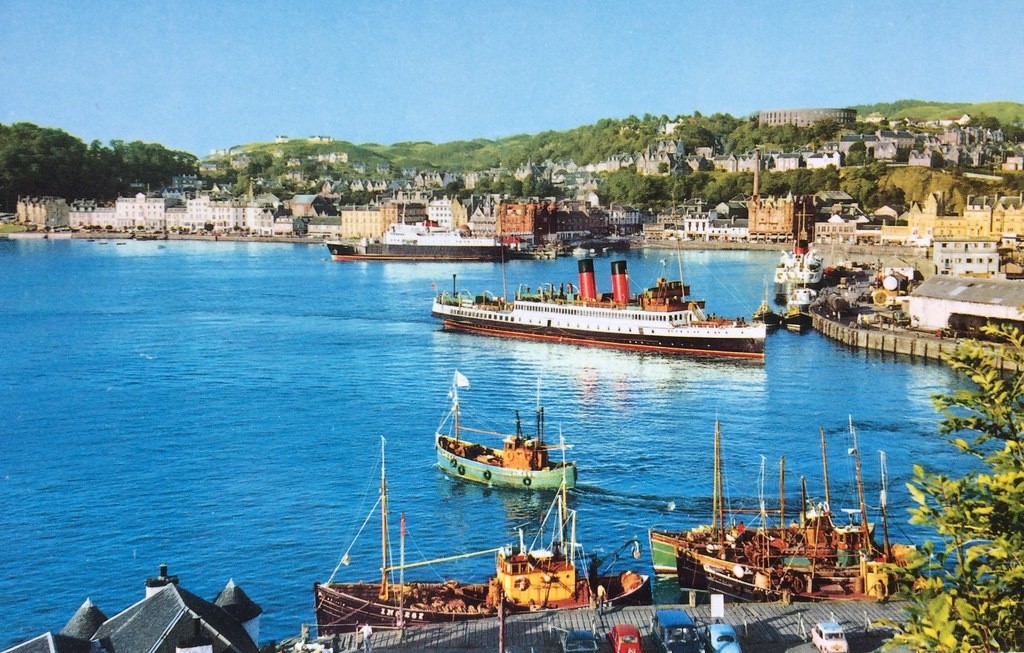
xmin=357 ymin=621 xmax=373 ymax=653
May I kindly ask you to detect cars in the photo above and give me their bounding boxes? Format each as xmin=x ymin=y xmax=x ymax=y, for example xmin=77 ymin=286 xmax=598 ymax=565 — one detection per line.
xmin=837 ymin=284 xmax=847 ymax=289
xmin=811 ymin=621 xmax=848 ymax=653
xmin=667 ymin=236 xmax=678 ymax=241
xmin=704 ymin=623 xmax=742 ymax=653
xmin=681 ymin=237 xmax=693 ymax=242
xmin=558 ymin=629 xmax=599 ymax=653
xmin=608 ymin=624 xmax=642 ymax=653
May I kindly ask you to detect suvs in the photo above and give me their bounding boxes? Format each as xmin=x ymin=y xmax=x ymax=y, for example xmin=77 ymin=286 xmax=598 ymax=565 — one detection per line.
xmin=651 ymin=608 xmax=706 ymax=653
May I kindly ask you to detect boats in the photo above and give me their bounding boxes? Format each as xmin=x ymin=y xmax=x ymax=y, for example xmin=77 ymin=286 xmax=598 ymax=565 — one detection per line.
xmin=572 ymin=247 xmax=589 ymax=255
xmin=783 ymin=306 xmax=813 ymax=334
xmin=430 ymin=188 xmax=768 ymax=366
xmin=87 ymin=238 xmax=94 ymax=242
xmin=774 ymin=196 xmax=826 ymax=299
xmin=323 ymin=200 xmax=510 ymax=262
xmin=99 ymin=240 xmax=108 ymax=243
xmin=433 ymin=364 xmax=579 ymax=493
xmin=785 ymin=266 xmax=818 ymax=313
xmin=116 ymin=241 xmax=127 ymax=245
xmin=135 ymin=234 xmax=168 ymax=240
xmin=752 ymin=277 xmax=782 ymax=331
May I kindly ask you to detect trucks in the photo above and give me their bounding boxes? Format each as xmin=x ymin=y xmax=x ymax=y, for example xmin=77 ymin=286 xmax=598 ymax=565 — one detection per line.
xmin=885 ymin=296 xmax=910 ymax=311
xmin=877 ymin=310 xmax=909 ymax=326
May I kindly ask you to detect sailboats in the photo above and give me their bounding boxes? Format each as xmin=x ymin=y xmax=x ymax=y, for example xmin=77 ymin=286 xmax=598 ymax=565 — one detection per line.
xmin=313 ymin=434 xmax=651 ymax=639
xmin=648 ymin=404 xmax=931 ymax=604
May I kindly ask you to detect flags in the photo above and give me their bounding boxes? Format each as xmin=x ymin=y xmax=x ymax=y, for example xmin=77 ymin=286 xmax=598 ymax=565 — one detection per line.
xmin=456 ymin=371 xmax=472 ymax=390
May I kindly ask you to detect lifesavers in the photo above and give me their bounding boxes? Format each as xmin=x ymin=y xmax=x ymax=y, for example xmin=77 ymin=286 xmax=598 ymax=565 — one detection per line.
xmin=731 ymin=583 xmax=743 ymax=595
xmin=514 ymin=576 xmax=528 ymax=591
xmin=523 ymin=477 xmax=532 ymax=486
xmin=750 ymin=590 xmax=765 ymax=603
xmin=449 ymin=459 xmax=457 ymax=468
xmin=483 ymin=470 xmax=492 ymax=481
xmin=766 ymin=592 xmax=778 ymax=603
xmin=458 ymin=465 xmax=465 ymax=475
